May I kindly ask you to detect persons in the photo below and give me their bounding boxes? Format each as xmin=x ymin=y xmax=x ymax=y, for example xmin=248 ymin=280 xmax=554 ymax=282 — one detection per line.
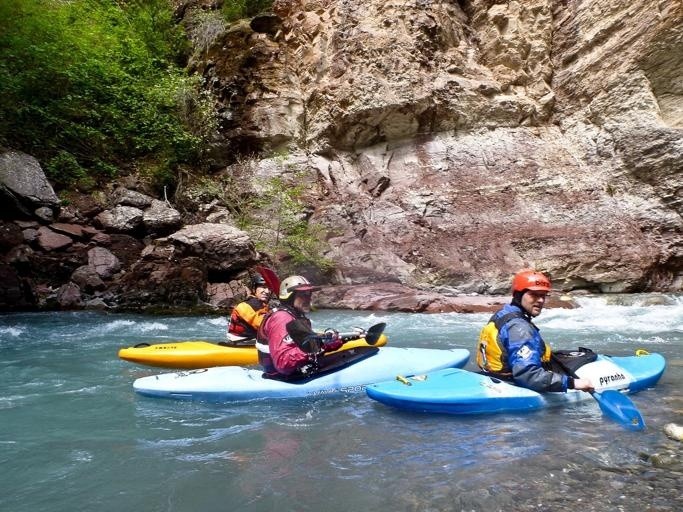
xmin=226 ymin=274 xmax=273 ymax=348
xmin=255 ymin=277 xmax=369 ymax=379
xmin=478 ymin=271 xmax=597 ymax=391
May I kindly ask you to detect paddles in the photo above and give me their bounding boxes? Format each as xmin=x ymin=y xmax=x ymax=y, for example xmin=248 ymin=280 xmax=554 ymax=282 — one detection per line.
xmin=552 ymin=351 xmax=644 ymax=432
xmin=310 ymin=322 xmax=386 ymax=347
xmin=258 ymin=264 xmax=282 ymax=294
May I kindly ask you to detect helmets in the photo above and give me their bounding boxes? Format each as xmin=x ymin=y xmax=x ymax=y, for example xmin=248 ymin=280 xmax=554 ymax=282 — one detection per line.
xmin=279 ymin=276 xmax=321 ymax=301
xmin=512 ymin=271 xmax=551 ymax=296
xmin=251 ymin=274 xmax=266 ymax=285
